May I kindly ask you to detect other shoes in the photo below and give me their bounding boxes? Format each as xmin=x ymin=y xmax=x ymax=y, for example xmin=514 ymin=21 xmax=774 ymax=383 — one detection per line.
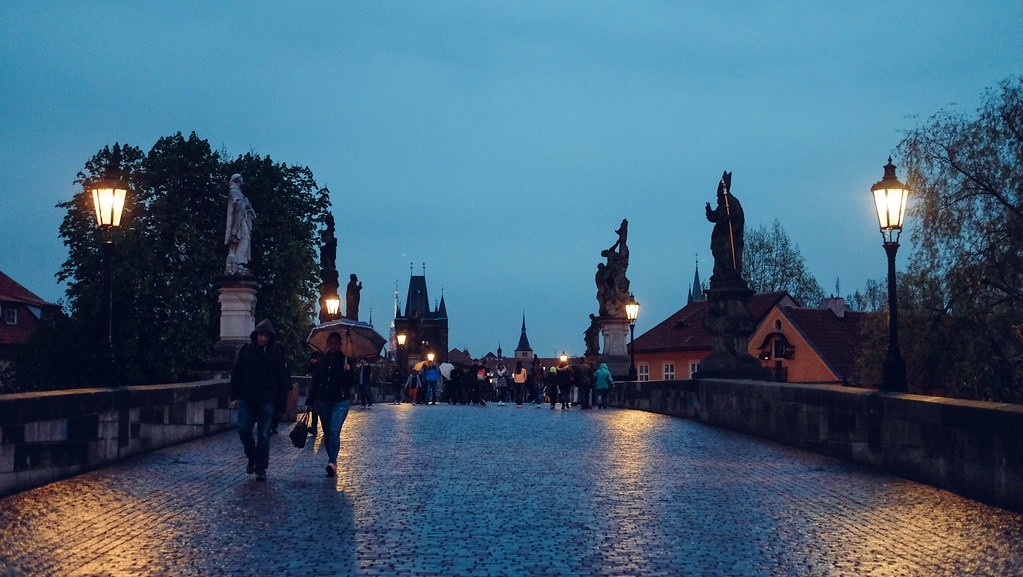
xmin=367 ymin=404 xmax=374 ymax=409
xmin=326 ymin=463 xmax=334 ymax=476
xmin=307 ymin=426 xmax=318 ymax=435
xmin=359 ymin=405 xmax=366 ymax=409
xmin=255 ymin=466 xmax=267 ymax=481
xmin=246 ymin=460 xmax=254 ymax=474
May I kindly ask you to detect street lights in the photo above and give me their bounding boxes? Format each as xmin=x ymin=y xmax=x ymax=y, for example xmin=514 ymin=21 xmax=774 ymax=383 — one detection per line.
xmin=623 ymin=290 xmax=641 ymax=382
xmin=871 ymin=157 xmax=914 ymax=393
xmin=87 ymin=175 xmax=130 ymax=388
xmin=396 ymin=330 xmax=407 ymax=402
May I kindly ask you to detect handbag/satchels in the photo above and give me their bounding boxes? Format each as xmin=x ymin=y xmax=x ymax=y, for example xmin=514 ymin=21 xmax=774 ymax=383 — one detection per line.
xmin=289 ymin=410 xmax=310 ymax=449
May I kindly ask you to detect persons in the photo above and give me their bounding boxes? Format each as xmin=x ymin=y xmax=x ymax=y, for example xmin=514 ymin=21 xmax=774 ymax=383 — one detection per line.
xmin=307 ymin=351 xmax=322 ymax=435
xmin=224 ymin=174 xmax=257 ymax=276
xmin=356 ymin=357 xmax=374 ymax=409
xmin=305 ymin=331 xmax=356 ymax=475
xmin=270 ymin=340 xmax=294 ymax=434
xmin=391 ymin=355 xmax=615 ymax=409
xmin=582 ymin=236 xmax=626 ymax=355
xmin=346 ymin=274 xmax=362 ymax=316
xmin=228 ymin=319 xmax=285 ymax=481
xmin=705 ymin=190 xmax=744 ymax=281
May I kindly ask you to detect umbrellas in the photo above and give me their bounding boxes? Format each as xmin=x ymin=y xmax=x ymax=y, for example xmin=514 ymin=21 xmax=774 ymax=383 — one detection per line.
xmin=305 ymin=318 xmax=388 ymax=367
xmin=357 ymin=354 xmax=388 ymax=366
xmin=438 ymin=362 xmax=456 ymax=381
